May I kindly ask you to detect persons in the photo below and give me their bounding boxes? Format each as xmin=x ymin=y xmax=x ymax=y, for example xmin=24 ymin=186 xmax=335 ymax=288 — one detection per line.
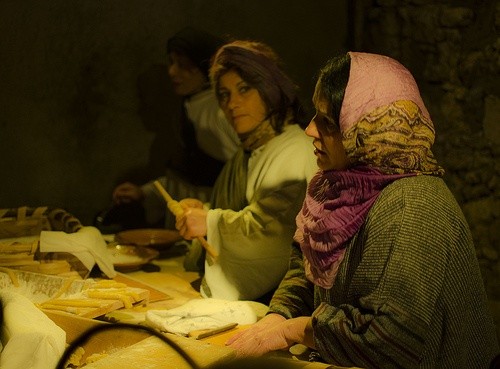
xmin=171 ymin=39 xmax=319 ymax=304
xmin=110 ymin=29 xmax=242 ymax=206
xmin=224 ymin=51 xmax=497 ymax=369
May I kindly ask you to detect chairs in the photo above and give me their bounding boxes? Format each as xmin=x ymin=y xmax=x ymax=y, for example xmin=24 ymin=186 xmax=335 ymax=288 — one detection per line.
xmin=0 ymin=206 xmax=88 ymax=238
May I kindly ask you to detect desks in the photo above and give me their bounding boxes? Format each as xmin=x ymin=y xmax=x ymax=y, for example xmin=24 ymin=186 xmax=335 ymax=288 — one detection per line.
xmin=0 ymin=233 xmax=299 ymax=369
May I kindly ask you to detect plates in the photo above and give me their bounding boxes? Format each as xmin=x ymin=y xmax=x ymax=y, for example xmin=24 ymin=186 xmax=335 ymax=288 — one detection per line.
xmin=105 ymin=242 xmax=160 ymax=272
xmin=117 ymin=226 xmax=180 ymax=251
